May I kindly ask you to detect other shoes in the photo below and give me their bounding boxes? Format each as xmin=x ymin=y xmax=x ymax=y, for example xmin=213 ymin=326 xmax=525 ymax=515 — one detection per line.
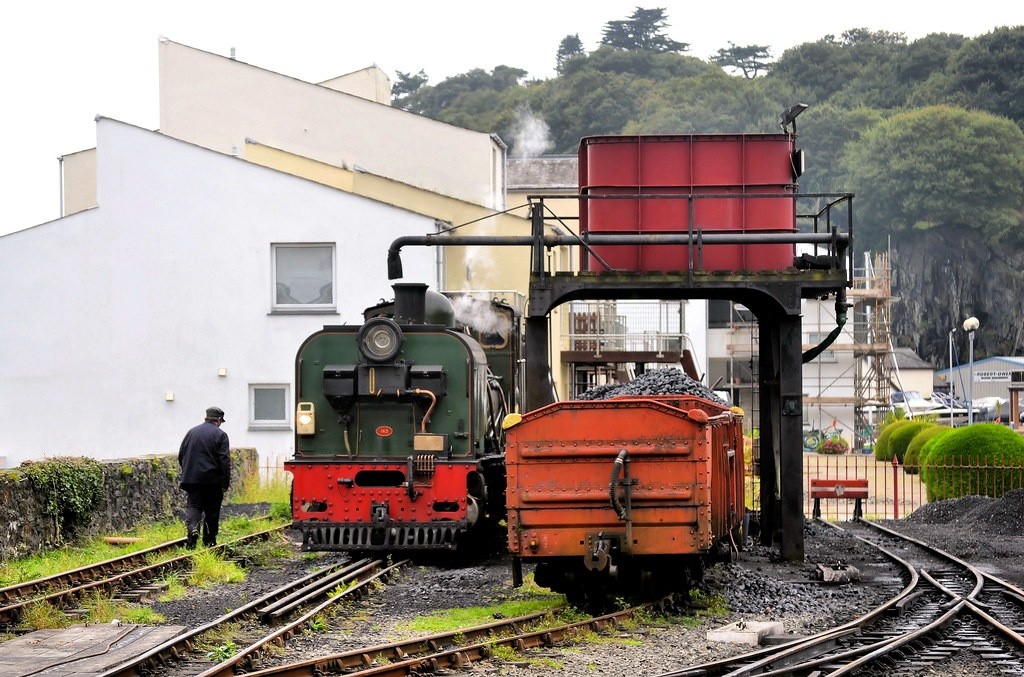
xmin=186 ymin=529 xmax=200 ymax=550
xmin=203 ymin=536 xmax=216 ymax=548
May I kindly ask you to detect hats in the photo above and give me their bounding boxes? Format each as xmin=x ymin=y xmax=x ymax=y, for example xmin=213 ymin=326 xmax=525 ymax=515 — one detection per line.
xmin=205 ymin=406 xmax=226 ymax=422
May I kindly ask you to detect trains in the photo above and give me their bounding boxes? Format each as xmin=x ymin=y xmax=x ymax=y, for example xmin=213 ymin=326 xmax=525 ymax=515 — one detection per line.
xmin=283 ymin=282 xmax=527 ymax=551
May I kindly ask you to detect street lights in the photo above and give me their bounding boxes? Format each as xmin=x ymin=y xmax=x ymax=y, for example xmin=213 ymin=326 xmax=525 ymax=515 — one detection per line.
xmin=963 ymin=317 xmax=979 ymax=426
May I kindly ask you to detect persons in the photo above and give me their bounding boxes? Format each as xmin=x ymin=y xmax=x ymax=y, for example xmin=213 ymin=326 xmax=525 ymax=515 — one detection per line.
xmin=178 ymin=407 xmax=230 ymax=550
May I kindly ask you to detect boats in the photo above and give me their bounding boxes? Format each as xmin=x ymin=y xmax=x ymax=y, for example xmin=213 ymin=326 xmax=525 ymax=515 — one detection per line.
xmin=857 ymin=391 xmax=980 ymax=427
